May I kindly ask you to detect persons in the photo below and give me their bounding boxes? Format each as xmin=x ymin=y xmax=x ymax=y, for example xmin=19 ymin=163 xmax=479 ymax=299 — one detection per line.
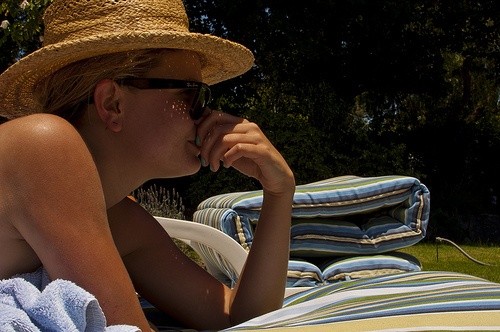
xmin=0 ymin=0 xmax=295 ymax=332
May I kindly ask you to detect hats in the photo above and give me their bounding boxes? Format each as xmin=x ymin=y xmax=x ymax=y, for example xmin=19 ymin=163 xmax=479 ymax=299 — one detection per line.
xmin=0 ymin=0 xmax=255 ymax=120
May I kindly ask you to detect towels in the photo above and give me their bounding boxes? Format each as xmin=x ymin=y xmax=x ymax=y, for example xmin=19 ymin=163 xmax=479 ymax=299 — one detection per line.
xmin=0 ymin=267 xmax=139 ymax=332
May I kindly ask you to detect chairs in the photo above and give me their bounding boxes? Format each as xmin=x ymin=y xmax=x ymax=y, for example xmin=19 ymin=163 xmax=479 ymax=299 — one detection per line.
xmin=154 ymin=216 xmax=314 ymax=299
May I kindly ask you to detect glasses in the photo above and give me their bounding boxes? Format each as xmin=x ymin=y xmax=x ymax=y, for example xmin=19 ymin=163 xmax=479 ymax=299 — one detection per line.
xmin=113 ymin=76 xmax=211 ymax=122
